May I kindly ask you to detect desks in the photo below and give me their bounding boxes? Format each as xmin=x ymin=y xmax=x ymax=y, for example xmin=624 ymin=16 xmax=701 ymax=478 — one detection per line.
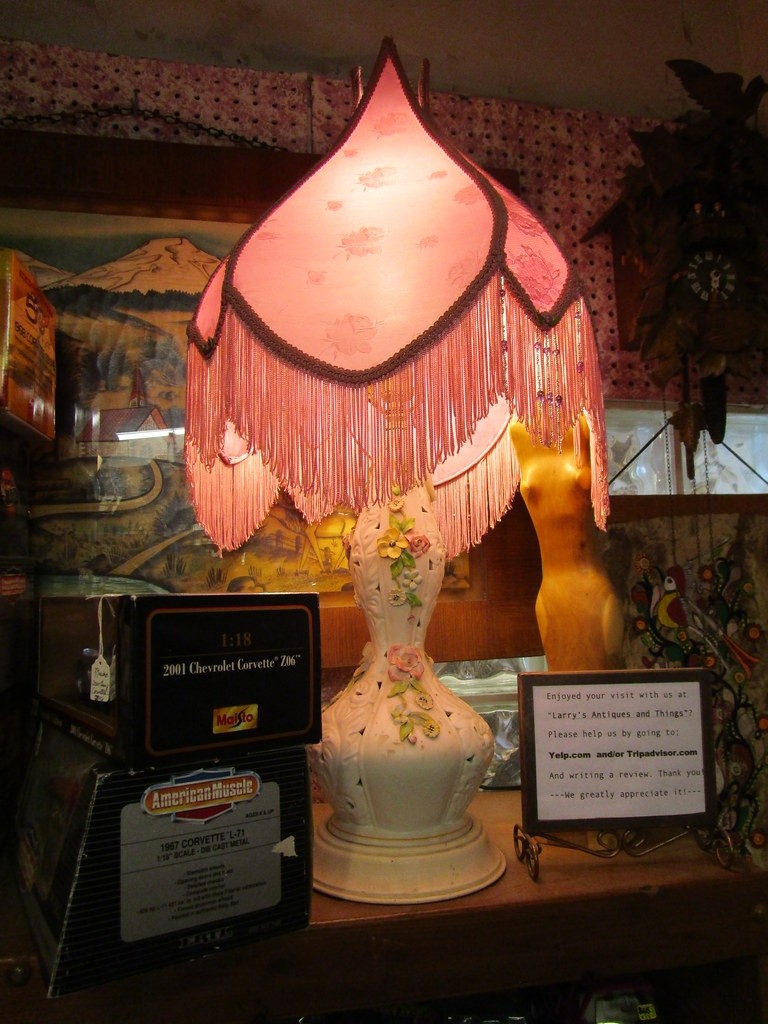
xmin=0 ymin=788 xmax=763 ymax=1024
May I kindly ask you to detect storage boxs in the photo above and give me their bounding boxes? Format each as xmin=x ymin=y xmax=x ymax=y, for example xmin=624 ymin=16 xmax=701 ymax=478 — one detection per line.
xmin=16 ymin=593 xmax=314 ymax=1001
xmin=0 ymin=248 xmax=55 ymax=847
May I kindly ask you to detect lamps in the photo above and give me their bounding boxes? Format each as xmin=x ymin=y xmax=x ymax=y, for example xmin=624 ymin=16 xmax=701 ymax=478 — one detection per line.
xmin=182 ymin=40 xmax=618 ymax=905
